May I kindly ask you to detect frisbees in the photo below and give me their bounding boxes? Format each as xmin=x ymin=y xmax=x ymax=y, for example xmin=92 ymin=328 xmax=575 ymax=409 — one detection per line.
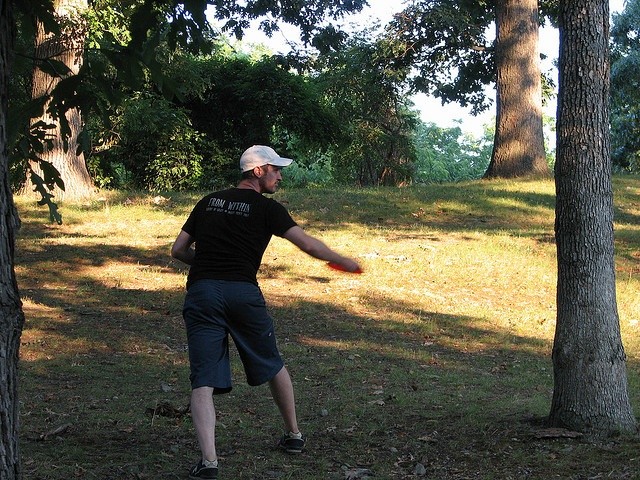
xmin=326 ymin=262 xmax=363 ymax=273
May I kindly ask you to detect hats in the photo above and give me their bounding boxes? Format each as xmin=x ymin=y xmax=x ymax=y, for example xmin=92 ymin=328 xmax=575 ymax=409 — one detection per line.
xmin=240 ymin=145 xmax=293 ymax=173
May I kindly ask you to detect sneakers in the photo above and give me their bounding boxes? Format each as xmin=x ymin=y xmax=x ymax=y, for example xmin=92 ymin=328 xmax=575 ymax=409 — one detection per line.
xmin=189 ymin=459 xmax=218 ymax=480
xmin=278 ymin=430 xmax=305 ymax=452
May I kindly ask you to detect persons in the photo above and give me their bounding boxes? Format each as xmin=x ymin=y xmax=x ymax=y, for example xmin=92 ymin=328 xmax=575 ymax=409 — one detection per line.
xmin=171 ymin=145 xmax=359 ymax=480
